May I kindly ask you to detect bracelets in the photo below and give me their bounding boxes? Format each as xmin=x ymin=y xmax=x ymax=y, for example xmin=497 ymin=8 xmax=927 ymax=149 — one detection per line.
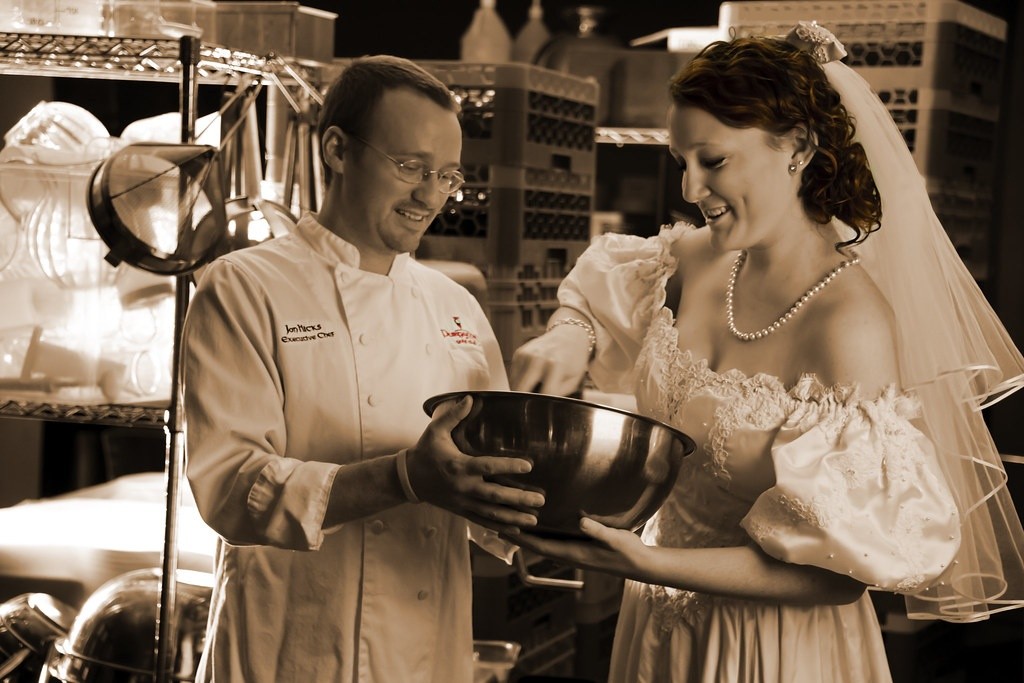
xmin=546 ymin=317 xmax=597 ymax=357
xmin=396 ymin=446 xmax=425 ymax=505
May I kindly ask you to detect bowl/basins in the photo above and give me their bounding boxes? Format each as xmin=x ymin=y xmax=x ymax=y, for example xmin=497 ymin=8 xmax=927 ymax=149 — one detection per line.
xmin=422 ymin=390 xmax=697 ymax=545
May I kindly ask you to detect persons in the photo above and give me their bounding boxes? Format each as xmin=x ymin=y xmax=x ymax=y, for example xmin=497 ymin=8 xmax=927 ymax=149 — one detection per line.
xmin=508 ymin=19 xmax=1024 ymax=683
xmin=177 ymin=54 xmax=545 ymax=683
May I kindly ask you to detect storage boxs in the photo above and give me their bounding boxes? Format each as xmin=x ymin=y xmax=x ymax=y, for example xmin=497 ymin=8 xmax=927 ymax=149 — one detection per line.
xmin=479 ymin=268 xmax=573 ymax=374
xmin=718 ymin=0 xmax=1007 ymax=301
xmin=413 ymin=62 xmax=599 ymax=174
xmin=410 ymin=166 xmax=595 ymax=268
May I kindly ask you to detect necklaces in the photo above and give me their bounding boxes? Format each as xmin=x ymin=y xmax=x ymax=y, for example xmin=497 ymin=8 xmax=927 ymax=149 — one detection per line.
xmin=727 ymin=248 xmax=860 ymax=341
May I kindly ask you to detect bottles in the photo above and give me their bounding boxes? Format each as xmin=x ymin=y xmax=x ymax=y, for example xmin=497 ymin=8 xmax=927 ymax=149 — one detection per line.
xmin=514 ymin=0 xmax=551 ymax=63
xmin=462 ymin=0 xmax=512 ymax=64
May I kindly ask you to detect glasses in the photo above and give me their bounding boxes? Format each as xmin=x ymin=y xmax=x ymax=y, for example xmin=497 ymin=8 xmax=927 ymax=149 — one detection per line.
xmin=356 ymin=141 xmax=465 ymax=194
